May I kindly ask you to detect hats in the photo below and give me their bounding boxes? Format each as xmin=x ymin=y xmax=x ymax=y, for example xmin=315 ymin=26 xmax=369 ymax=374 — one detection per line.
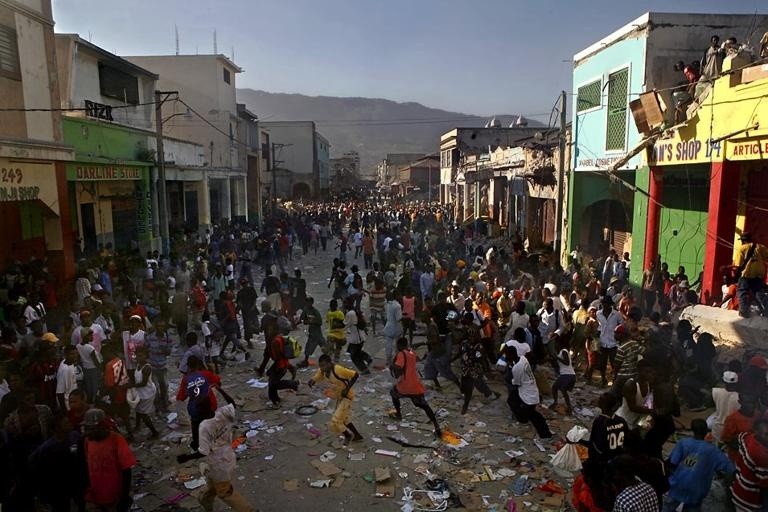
xmin=600 ymin=295 xmax=614 ymax=304
xmin=738 ymin=232 xmax=752 ymax=240
xmin=722 ymin=371 xmax=738 ymax=383
xmin=79 ymin=408 xmax=105 ymax=426
xmin=685 ymin=419 xmax=711 ymax=433
xmin=678 ymin=279 xmax=689 ymax=288
xmin=609 ymin=276 xmax=621 ymax=287
xmin=749 ymin=356 xmax=767 ymax=369
xmin=39 ymin=332 xmax=59 ymax=343
xmin=240 ymin=278 xmax=250 ymax=285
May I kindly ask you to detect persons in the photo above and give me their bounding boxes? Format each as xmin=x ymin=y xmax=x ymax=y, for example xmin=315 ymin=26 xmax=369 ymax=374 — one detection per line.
xmin=1 ymin=184 xmax=767 ymax=511
xmin=671 ymin=28 xmax=768 ymax=127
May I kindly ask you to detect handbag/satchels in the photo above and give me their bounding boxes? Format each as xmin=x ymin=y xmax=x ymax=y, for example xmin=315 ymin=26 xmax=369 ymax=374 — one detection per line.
xmin=389 ymin=351 xmax=406 ymax=379
xmin=95 ymin=387 xmax=115 ymax=407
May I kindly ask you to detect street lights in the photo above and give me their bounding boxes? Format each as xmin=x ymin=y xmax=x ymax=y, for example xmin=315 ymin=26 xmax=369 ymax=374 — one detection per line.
xmin=150 ymin=109 xmax=202 ymax=266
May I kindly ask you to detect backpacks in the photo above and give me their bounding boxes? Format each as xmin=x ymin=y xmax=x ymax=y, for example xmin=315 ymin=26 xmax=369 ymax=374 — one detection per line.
xmin=267 ymin=313 xmax=290 ymax=335
xmin=354 ymin=307 xmax=369 ymax=343
xmin=352 ymin=272 xmax=363 ymax=289
xmin=192 ymin=288 xmax=206 ymax=310
xmin=282 ymin=335 xmax=302 ymax=359
xmin=207 ymin=319 xmax=224 ymax=341
